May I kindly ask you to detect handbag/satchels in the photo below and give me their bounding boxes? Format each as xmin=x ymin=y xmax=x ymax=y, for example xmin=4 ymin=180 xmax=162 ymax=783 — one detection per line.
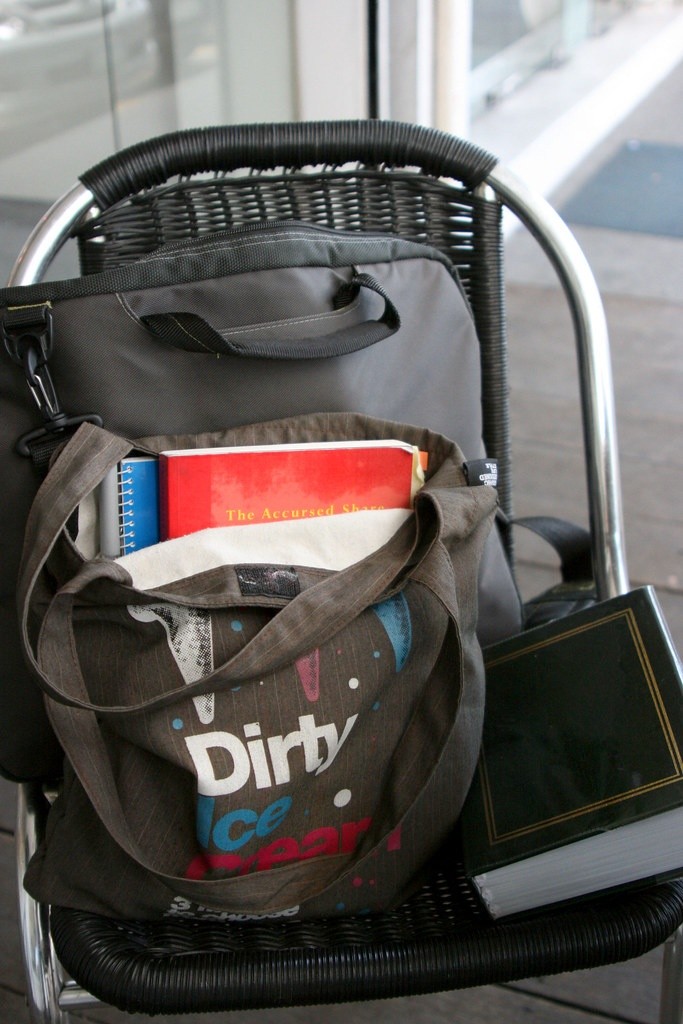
xmin=14 ymin=411 xmax=499 ymax=925
xmin=0 ymin=220 xmax=525 ymax=785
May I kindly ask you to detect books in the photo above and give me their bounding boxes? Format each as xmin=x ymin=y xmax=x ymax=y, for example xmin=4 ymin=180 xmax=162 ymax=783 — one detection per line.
xmin=465 ymin=585 xmax=683 ymax=920
xmin=111 ymin=439 xmax=419 ymax=558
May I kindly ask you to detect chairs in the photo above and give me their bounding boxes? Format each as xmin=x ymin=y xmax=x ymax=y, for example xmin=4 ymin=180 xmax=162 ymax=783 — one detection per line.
xmin=16 ymin=120 xmax=683 ymax=1023
